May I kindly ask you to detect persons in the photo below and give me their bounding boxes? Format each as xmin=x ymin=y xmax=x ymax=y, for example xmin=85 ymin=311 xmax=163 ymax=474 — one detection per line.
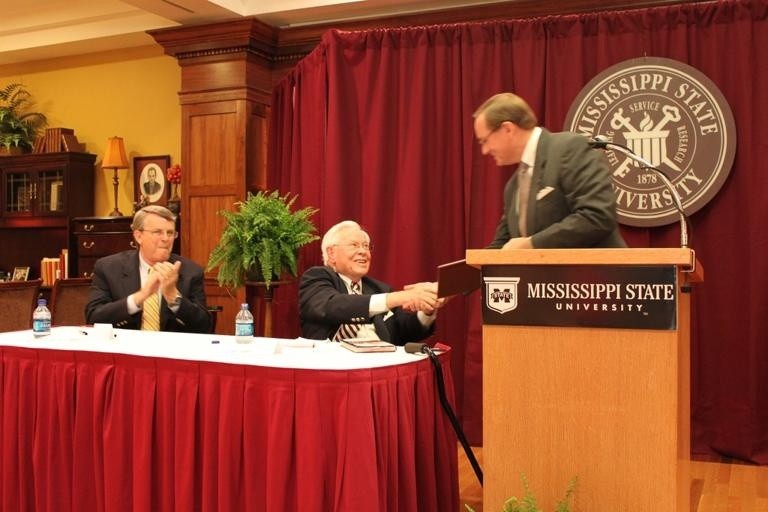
xmin=144 ymin=169 xmax=161 ymax=195
xmin=403 ymin=93 xmax=628 ymax=311
xmin=299 ymin=220 xmax=445 ymax=346
xmin=85 ymin=205 xmax=211 ymax=333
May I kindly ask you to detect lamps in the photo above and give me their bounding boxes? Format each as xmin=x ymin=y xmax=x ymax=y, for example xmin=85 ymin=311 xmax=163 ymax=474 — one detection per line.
xmin=101 ymin=135 xmax=130 ymax=216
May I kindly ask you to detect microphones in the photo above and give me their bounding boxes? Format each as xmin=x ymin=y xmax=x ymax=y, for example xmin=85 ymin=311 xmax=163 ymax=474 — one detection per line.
xmin=588 ymin=134 xmax=689 ymax=248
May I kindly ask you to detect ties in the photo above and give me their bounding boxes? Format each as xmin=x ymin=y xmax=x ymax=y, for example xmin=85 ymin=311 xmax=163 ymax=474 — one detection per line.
xmin=332 ymin=283 xmax=361 ymax=342
xmin=517 ymin=163 xmax=531 ymax=239
xmin=141 ymin=269 xmax=161 ymax=332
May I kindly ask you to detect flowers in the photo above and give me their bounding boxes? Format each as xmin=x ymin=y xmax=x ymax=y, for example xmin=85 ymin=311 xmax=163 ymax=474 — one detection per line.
xmin=167 ymin=164 xmax=181 ymax=200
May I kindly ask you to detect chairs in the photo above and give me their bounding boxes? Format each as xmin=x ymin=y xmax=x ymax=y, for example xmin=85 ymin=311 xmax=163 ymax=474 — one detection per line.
xmin=0 ymin=278 xmax=44 ymax=332
xmin=50 ymin=278 xmax=94 ymax=327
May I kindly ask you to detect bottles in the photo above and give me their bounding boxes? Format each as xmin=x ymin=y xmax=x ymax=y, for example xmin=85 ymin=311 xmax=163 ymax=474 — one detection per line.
xmin=236 ymin=302 xmax=255 ymax=361
xmin=32 ymin=299 xmax=52 ymax=339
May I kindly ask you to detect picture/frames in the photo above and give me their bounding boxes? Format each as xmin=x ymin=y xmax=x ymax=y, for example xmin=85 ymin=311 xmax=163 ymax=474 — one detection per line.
xmin=11 ymin=266 xmax=31 ymax=281
xmin=132 ymin=155 xmax=170 ymax=212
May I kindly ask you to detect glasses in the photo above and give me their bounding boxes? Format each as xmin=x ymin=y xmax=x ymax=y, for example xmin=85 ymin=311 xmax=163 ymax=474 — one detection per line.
xmin=480 ymin=122 xmax=501 ymax=145
xmin=332 ymin=242 xmax=376 ymax=252
xmin=139 ymin=229 xmax=179 ymax=240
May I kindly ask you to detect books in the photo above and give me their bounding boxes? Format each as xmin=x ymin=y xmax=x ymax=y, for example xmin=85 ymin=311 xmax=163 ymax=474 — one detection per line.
xmin=17 ymin=182 xmax=64 ymax=212
xmin=32 ymin=128 xmax=81 ymax=154
xmin=437 ymin=259 xmax=481 ymax=299
xmin=41 ymin=249 xmax=68 ymax=287
xmin=340 ymin=340 xmax=396 ymax=353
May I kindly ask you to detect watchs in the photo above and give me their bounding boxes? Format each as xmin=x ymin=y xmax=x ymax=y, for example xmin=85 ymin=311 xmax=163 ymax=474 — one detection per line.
xmin=169 ymin=292 xmax=183 ymax=308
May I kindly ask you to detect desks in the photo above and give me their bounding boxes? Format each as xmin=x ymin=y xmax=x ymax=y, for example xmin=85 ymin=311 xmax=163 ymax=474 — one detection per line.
xmin=0 ymin=322 xmax=461 ymax=512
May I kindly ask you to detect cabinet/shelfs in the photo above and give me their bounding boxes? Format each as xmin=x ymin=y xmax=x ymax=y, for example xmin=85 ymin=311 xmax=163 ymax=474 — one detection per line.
xmin=71 ymin=217 xmax=138 ymax=278
xmin=0 ymin=152 xmax=97 ymax=228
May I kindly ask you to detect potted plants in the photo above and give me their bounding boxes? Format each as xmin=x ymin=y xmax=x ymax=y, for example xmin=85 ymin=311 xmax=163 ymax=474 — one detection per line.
xmin=204 ymin=188 xmax=321 ymax=303
xmin=0 ymin=81 xmax=49 ymax=155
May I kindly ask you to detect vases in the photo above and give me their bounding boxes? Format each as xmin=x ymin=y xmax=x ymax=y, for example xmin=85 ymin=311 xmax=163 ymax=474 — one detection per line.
xmin=168 ymin=200 xmax=180 ymax=213
